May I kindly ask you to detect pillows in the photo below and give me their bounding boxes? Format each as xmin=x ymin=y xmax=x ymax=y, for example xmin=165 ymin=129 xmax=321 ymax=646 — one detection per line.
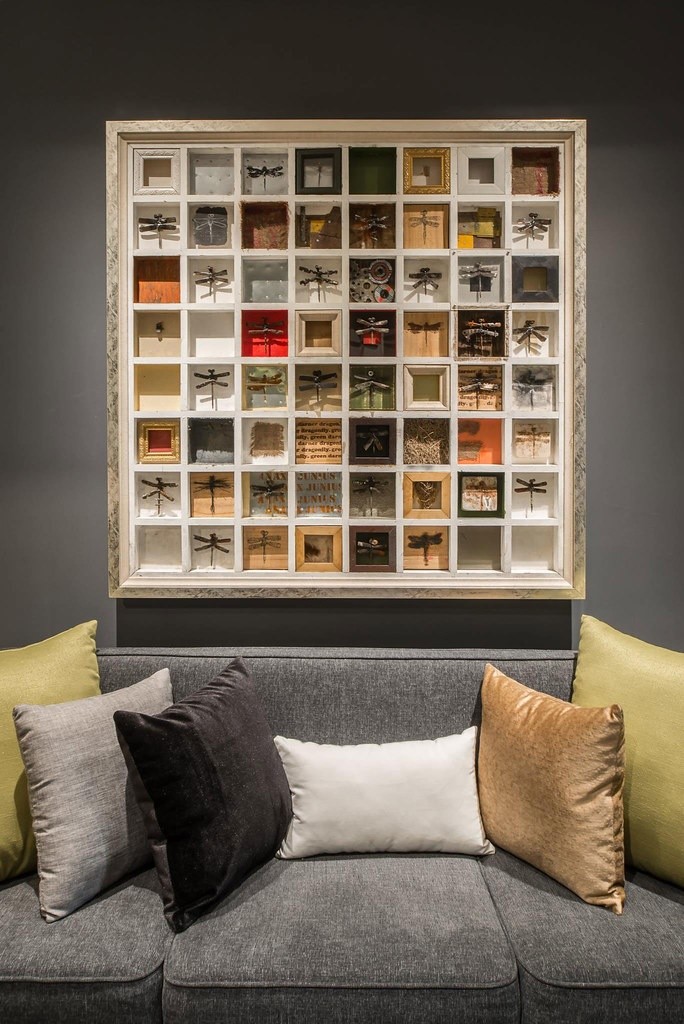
xmin=274 ymin=727 xmax=497 ymax=859
xmin=115 ymin=654 xmax=293 ymax=935
xmin=480 ymin=662 xmax=626 ymax=915
xmin=0 ymin=619 xmax=101 ymax=883
xmin=13 ymin=668 xmax=174 ymax=923
xmin=572 ymin=615 xmax=684 ymax=888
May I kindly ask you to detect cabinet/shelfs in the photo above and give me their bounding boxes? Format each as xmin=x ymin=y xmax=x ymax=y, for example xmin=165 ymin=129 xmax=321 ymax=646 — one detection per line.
xmin=105 ymin=121 xmax=584 ymax=599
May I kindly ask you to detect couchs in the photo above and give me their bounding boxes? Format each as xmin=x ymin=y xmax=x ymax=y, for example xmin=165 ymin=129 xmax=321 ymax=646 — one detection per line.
xmin=0 ymin=646 xmax=683 ymax=1024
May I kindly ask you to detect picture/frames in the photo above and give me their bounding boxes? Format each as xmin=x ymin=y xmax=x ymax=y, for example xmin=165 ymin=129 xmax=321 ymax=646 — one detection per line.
xmin=404 ymin=472 xmax=450 ymax=520
xmin=403 ymin=365 xmax=450 ymax=410
xmin=458 ymin=147 xmax=505 ymax=195
xmin=295 ymin=310 xmax=342 ymax=357
xmin=134 ymin=148 xmax=181 ymax=195
xmin=403 ymin=147 xmax=449 ymax=194
xmin=136 ymin=418 xmax=181 ymax=464
xmin=296 ymin=526 xmax=343 ymax=572
